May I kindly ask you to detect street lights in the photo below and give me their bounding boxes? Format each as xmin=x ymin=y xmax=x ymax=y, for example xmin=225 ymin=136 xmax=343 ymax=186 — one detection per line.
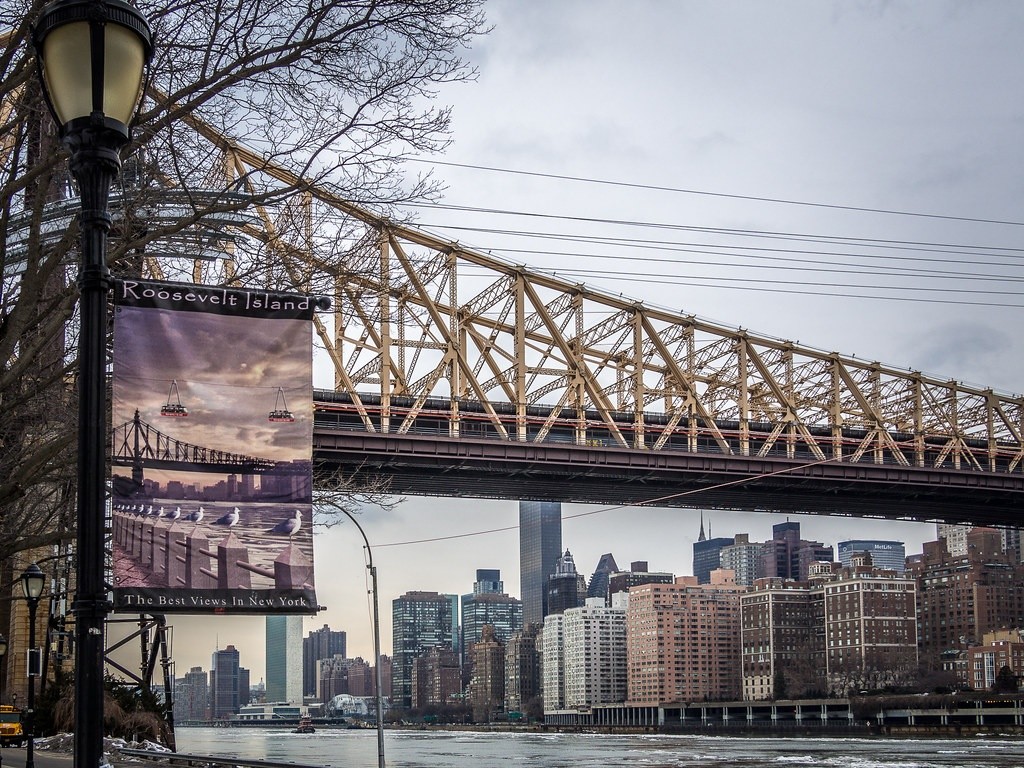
xmin=19 ymin=563 xmax=44 ymax=768
xmin=30 ymin=1 xmax=153 ymax=768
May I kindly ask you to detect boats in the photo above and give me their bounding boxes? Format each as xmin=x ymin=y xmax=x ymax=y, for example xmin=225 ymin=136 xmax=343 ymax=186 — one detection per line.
xmin=297 ymin=714 xmax=315 ymax=733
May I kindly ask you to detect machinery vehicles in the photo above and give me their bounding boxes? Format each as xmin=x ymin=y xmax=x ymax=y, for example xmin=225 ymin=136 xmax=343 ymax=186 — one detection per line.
xmin=0 ymin=705 xmax=23 ymax=748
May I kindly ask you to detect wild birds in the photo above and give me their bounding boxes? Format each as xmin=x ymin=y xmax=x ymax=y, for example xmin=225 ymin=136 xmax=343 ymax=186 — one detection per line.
xmin=134 ymin=504 xmax=145 ymax=515
xmin=116 ymin=505 xmax=121 ymax=510
xmin=113 ymin=504 xmax=117 ymax=510
xmin=208 ymin=507 xmax=244 ymax=532
xmin=262 ymin=510 xmax=304 ymax=544
xmin=140 ymin=505 xmax=152 ymax=518
xmin=180 ymin=507 xmax=205 ymax=527
xmin=160 ymin=507 xmax=182 ymax=522
xmin=149 ymin=507 xmax=164 ymax=520
xmin=124 ymin=505 xmax=130 ymax=512
xmin=128 ymin=505 xmax=136 ymax=512
xmin=119 ymin=505 xmax=125 ymax=511
